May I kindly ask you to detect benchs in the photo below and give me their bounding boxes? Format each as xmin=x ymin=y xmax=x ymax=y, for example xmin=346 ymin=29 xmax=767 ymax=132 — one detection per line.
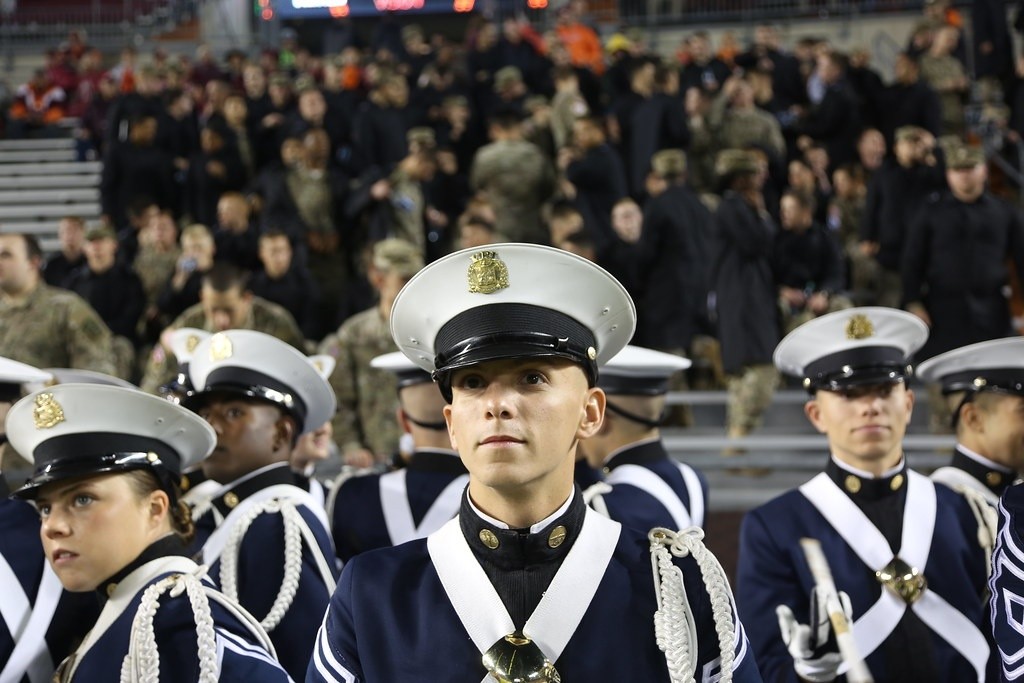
xmin=0 ymin=139 xmax=103 ymax=233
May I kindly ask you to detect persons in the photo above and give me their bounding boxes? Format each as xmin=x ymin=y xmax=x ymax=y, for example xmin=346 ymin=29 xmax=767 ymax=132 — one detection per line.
xmin=0 ymin=0 xmax=1024 ymax=683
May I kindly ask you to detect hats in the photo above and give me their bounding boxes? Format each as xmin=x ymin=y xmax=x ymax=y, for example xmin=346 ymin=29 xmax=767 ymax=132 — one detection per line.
xmin=772 ymin=306 xmax=929 ymax=391
xmin=42 ymin=367 xmax=146 ymax=388
xmin=309 ymin=354 xmax=336 ymax=381
xmin=729 ymin=152 xmax=764 ymax=174
xmin=370 ymin=350 xmax=433 ymax=390
xmin=179 ymin=328 xmax=337 ymax=429
xmin=390 ymin=242 xmax=637 ymax=404
xmin=157 ymin=328 xmax=215 ymax=398
xmin=594 ymin=344 xmax=692 ymax=395
xmin=374 ymin=238 xmax=423 ymax=276
xmin=945 ymin=143 xmax=985 ymax=168
xmin=916 ymin=336 xmax=1024 ymax=398
xmin=0 ymin=353 xmax=53 ymax=403
xmin=651 ymin=150 xmax=688 ymax=175
xmin=5 ymin=384 xmax=216 ymax=504
